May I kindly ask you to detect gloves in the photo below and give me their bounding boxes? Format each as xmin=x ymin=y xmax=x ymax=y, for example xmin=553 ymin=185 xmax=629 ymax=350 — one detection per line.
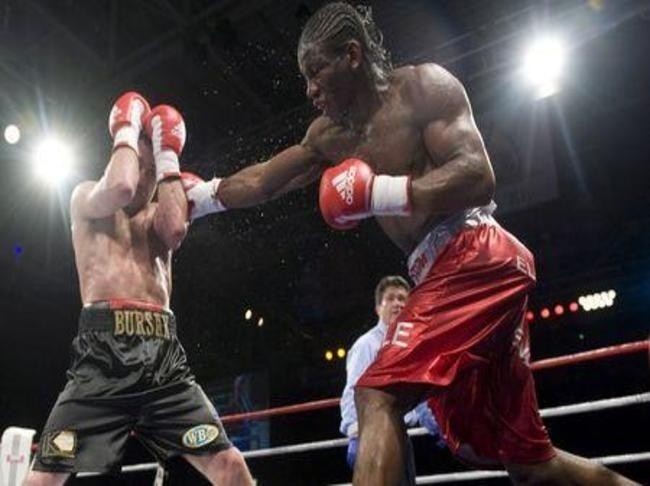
xmin=179 ymin=170 xmax=227 ymax=224
xmin=319 ymin=156 xmax=412 ymax=231
xmin=107 ymin=90 xmax=152 ymax=157
xmin=144 ymin=103 xmax=187 ymax=183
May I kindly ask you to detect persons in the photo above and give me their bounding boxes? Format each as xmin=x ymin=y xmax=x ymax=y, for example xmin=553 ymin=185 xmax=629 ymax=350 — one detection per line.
xmin=335 ymin=272 xmax=453 ymax=486
xmin=14 ymin=88 xmax=261 ymax=486
xmin=177 ymin=0 xmax=645 ymax=486
